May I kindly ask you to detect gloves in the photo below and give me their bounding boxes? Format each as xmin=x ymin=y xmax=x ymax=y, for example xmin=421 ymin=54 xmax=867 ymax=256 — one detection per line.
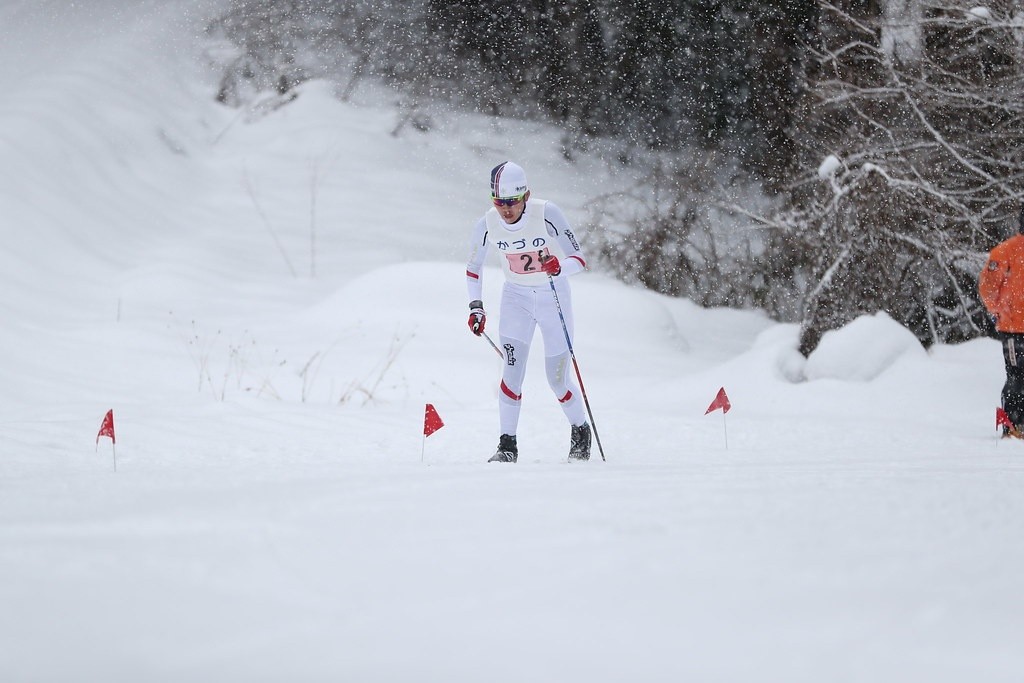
xmin=538 ymin=255 xmax=560 ymax=276
xmin=467 ymin=308 xmax=486 ymax=337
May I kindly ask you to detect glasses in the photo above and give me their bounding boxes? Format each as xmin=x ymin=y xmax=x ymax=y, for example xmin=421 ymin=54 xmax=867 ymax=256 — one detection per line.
xmin=491 ymin=192 xmax=524 ymax=207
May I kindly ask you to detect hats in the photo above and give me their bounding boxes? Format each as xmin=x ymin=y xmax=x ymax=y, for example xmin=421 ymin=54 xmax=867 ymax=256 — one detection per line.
xmin=489 ymin=161 xmax=528 ymax=197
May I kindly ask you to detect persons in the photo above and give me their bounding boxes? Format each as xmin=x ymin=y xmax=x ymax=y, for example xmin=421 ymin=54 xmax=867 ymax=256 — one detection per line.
xmin=466 ymin=161 xmax=592 ymax=465
xmin=980 ymin=202 xmax=1024 ymax=440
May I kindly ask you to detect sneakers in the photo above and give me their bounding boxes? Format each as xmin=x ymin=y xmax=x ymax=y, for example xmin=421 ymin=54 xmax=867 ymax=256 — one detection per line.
xmin=488 ymin=434 xmax=518 ymax=463
xmin=567 ymin=421 xmax=591 ymax=463
